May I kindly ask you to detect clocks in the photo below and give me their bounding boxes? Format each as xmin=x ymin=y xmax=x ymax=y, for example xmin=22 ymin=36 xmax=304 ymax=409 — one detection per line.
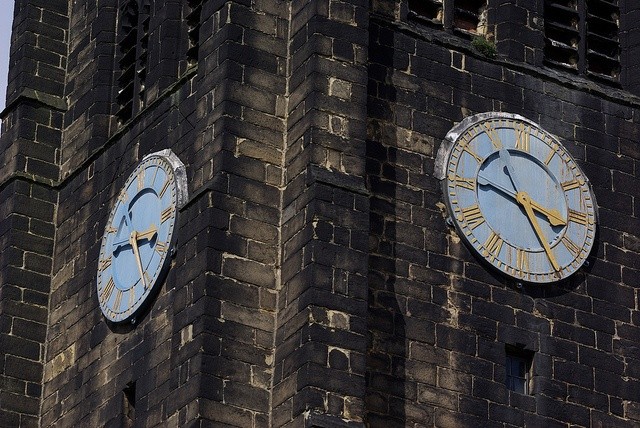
xmin=96 ymin=146 xmax=190 ymax=328
xmin=438 ymin=106 xmax=599 ymax=284
